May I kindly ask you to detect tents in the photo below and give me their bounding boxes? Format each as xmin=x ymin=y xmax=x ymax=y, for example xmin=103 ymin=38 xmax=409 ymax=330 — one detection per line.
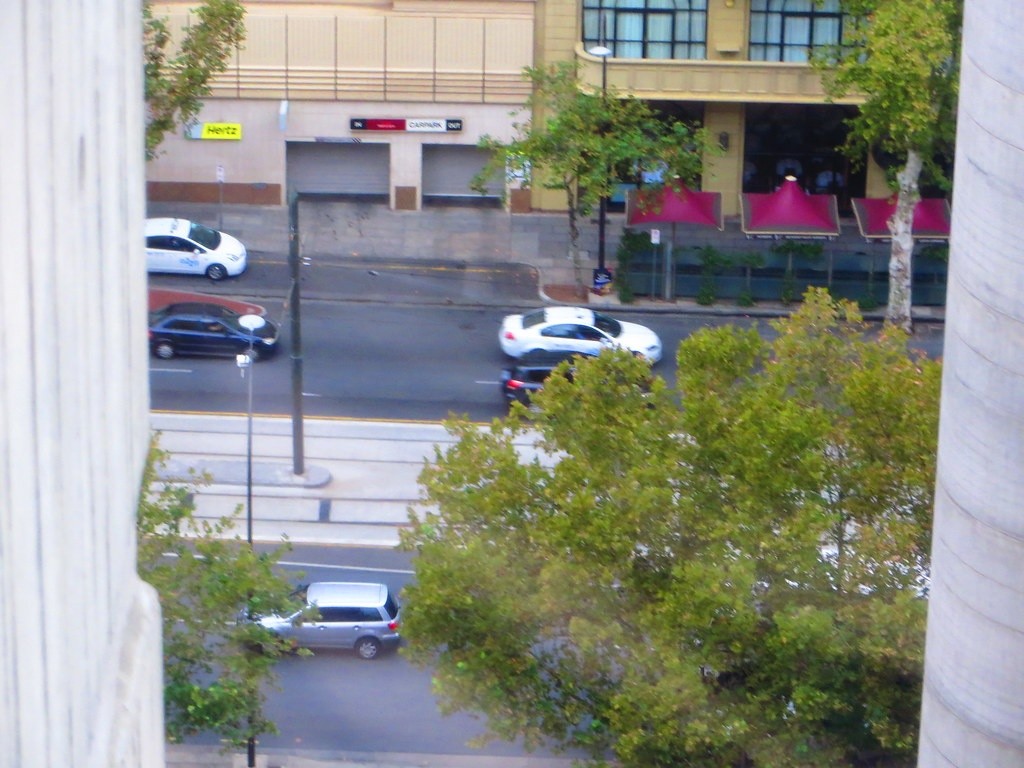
xmin=738 ymin=176 xmax=840 ymax=294
xmin=624 ymin=176 xmax=723 ymax=300
xmin=850 ymin=188 xmax=952 ymax=296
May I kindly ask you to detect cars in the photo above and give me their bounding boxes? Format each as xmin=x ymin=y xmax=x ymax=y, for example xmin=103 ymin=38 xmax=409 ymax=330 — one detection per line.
xmin=148 ymin=301 xmax=281 ymax=363
xmin=500 ymin=350 xmax=658 ymax=419
xmin=236 ymin=581 xmax=402 ymax=660
xmin=145 ymin=217 xmax=247 ymax=282
xmin=498 ymin=305 xmax=664 ymax=369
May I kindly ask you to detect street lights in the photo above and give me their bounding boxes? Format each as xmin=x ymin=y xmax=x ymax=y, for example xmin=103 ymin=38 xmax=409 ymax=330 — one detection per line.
xmin=235 ymin=313 xmax=267 ymax=546
xmin=587 ymin=44 xmax=613 ymax=273
